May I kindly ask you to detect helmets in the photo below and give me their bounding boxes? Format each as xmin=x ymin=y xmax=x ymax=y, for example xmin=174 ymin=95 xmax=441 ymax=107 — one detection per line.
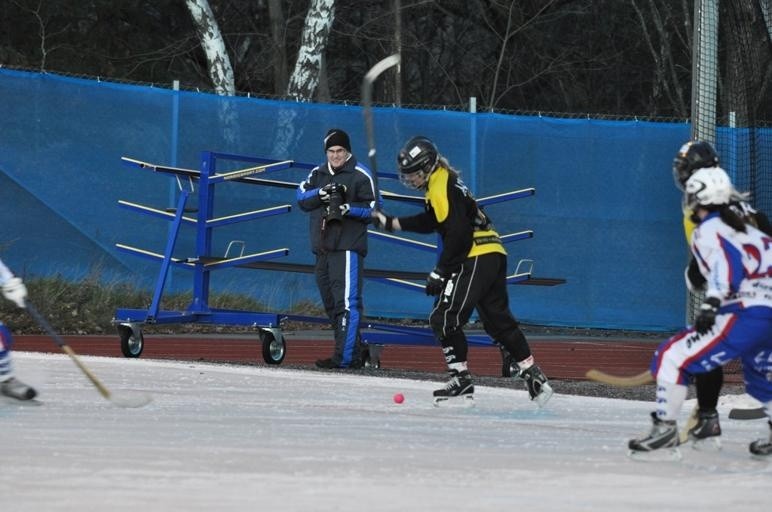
xmin=677 ymin=166 xmax=736 ymax=224
xmin=671 ymin=138 xmax=723 ymax=194
xmin=395 ymin=133 xmax=441 ymax=191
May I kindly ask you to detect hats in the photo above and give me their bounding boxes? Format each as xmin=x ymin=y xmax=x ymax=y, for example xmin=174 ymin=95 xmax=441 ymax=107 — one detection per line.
xmin=322 ymin=127 xmax=353 ymax=155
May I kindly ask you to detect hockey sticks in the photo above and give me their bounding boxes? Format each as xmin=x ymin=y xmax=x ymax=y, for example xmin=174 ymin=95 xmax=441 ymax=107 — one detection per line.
xmin=586 ymin=371 xmax=657 ymax=385
xmin=0 ymin=265 xmax=152 ymax=408
xmin=364 ymin=54 xmax=399 ymax=229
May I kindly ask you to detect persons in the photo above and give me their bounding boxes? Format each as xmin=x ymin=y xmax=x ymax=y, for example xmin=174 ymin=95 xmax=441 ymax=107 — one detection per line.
xmin=371 ymin=134 xmax=554 ymax=407
xmin=1 ymin=256 xmax=39 ymax=400
xmin=294 ymin=128 xmax=374 ymax=369
xmin=668 ymin=141 xmax=772 ymax=439
xmin=628 ymin=167 xmax=772 ymax=455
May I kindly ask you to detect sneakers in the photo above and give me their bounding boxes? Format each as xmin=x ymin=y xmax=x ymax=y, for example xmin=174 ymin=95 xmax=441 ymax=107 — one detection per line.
xmin=748 ymin=419 xmax=772 ymax=456
xmin=313 ymin=358 xmax=365 ymax=371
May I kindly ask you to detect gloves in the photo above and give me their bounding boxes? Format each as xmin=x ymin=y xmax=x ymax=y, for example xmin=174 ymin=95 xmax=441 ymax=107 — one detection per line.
xmin=0 ymin=274 xmax=30 ymax=312
xmin=319 ymin=182 xmax=349 ymax=205
xmin=422 ymin=265 xmax=452 ymax=297
xmin=369 ymin=209 xmax=404 ymax=234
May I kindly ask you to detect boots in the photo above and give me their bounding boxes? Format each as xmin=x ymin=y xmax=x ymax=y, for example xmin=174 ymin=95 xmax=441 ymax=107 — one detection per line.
xmin=1 ymin=377 xmax=41 ymax=402
xmin=433 ymin=365 xmax=476 ymax=397
xmin=684 ymin=407 xmax=723 ymax=442
xmin=516 ymin=362 xmax=547 ymax=401
xmin=626 ymin=410 xmax=681 ymax=453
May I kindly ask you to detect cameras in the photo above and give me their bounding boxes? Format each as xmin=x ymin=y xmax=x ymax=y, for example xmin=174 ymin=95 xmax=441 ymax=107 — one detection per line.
xmin=322 ymin=182 xmax=345 ymax=225
